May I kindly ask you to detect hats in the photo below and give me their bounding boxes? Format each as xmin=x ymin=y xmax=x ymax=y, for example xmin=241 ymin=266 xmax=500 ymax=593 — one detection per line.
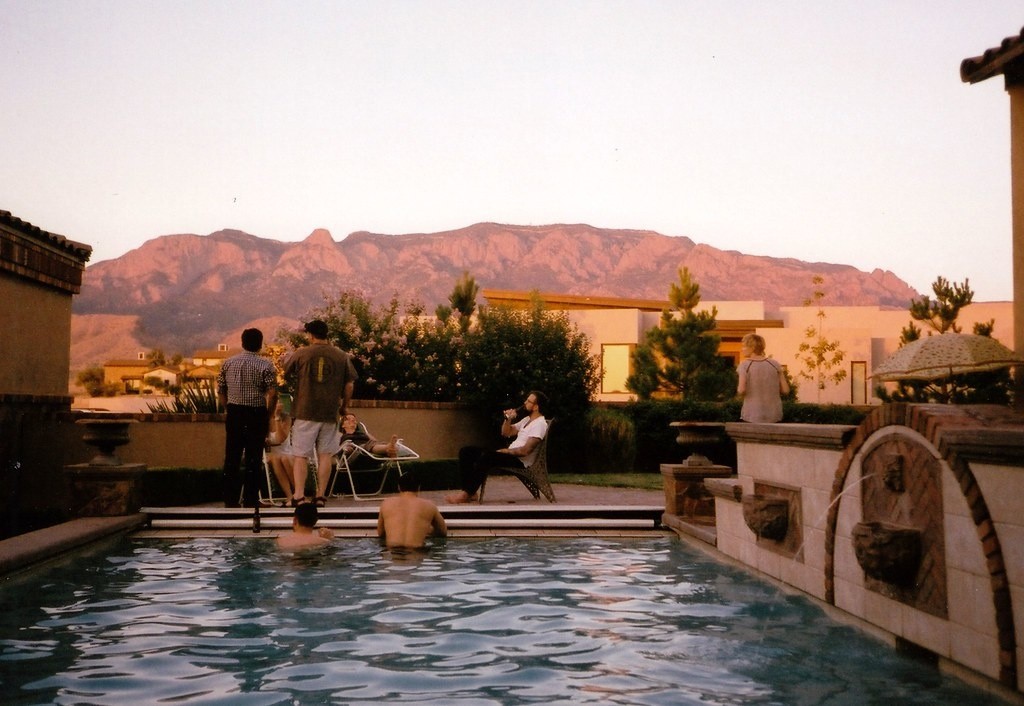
xmin=305 ymin=320 xmax=329 ymax=339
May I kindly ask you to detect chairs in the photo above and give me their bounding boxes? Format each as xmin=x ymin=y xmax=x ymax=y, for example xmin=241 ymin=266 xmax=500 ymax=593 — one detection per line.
xmin=477 ymin=415 xmax=556 ymax=504
xmin=239 ymin=418 xmax=419 ymax=506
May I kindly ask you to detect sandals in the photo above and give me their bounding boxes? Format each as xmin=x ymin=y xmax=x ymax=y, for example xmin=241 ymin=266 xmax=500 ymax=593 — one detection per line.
xmin=279 ymin=496 xmax=305 ymax=508
xmin=312 ymin=497 xmax=327 ymax=507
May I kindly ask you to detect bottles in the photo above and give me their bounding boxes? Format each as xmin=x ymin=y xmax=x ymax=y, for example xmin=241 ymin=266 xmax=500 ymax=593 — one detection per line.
xmin=504 ymin=405 xmax=526 ymax=420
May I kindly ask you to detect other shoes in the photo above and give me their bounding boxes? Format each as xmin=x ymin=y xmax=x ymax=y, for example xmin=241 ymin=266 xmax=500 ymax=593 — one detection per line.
xmin=243 ymin=501 xmax=271 ymax=508
xmin=224 ymin=503 xmax=241 ymax=508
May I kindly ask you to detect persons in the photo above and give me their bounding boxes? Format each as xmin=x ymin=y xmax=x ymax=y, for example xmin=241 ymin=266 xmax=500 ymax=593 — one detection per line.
xmin=445 ymin=390 xmax=547 ymax=504
xmin=267 ymin=399 xmax=295 ymax=504
xmin=377 ymin=471 xmax=447 ymax=548
xmin=735 ymin=333 xmax=790 ymax=422
xmin=276 ymin=504 xmax=332 ymax=554
xmin=338 ymin=413 xmax=398 ymax=458
xmin=216 ymin=328 xmax=278 ymax=508
xmin=282 ymin=320 xmax=359 ymax=507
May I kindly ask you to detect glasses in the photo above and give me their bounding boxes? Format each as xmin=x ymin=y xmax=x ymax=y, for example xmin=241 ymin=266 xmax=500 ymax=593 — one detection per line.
xmin=527 ymin=399 xmax=536 ymax=404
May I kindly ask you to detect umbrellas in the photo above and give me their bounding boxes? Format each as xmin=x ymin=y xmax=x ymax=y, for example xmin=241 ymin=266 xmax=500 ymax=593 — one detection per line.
xmin=866 ymin=333 xmax=1024 ymax=404
